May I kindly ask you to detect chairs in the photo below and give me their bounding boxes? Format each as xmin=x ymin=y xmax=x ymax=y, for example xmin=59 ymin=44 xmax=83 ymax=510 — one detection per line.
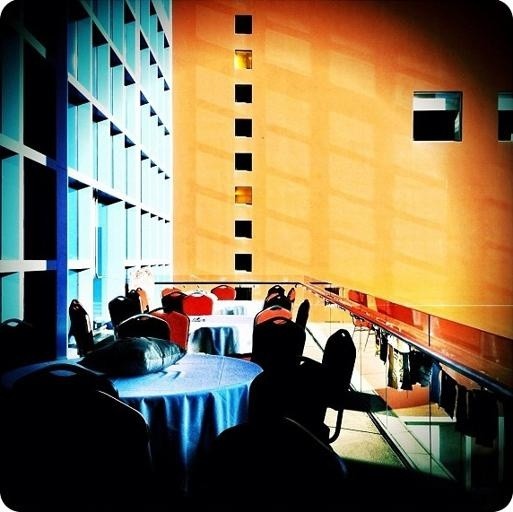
xmin=0 ymin=279 xmax=376 ymax=512
